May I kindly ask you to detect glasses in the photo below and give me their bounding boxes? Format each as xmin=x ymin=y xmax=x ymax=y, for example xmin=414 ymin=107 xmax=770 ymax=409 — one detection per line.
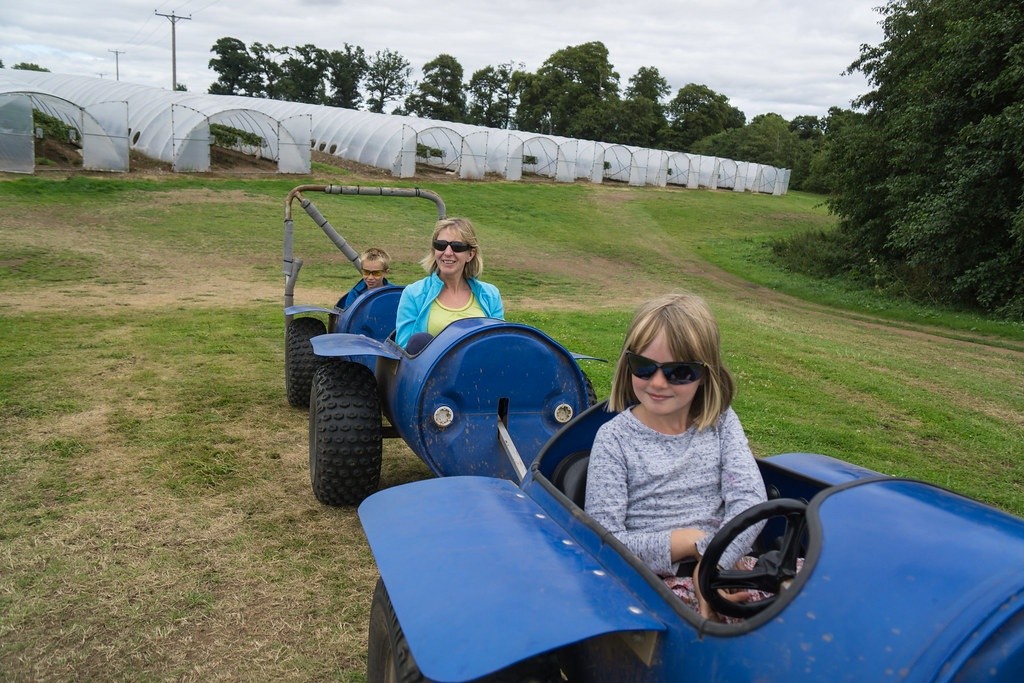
xmin=626 ymin=350 xmax=704 ymax=385
xmin=361 ymin=269 xmax=386 ymax=278
xmin=433 ymin=240 xmax=471 ymax=253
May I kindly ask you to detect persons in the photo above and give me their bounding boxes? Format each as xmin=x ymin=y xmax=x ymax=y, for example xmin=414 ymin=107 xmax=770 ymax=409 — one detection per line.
xmin=581 ymin=291 xmax=806 ymax=623
xmin=343 ymin=247 xmax=396 ymax=309
xmin=396 ymin=217 xmax=506 ymax=357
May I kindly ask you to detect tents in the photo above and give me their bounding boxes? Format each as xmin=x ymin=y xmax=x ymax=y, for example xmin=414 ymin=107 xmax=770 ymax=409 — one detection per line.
xmin=0 ymin=69 xmax=792 ymax=196
